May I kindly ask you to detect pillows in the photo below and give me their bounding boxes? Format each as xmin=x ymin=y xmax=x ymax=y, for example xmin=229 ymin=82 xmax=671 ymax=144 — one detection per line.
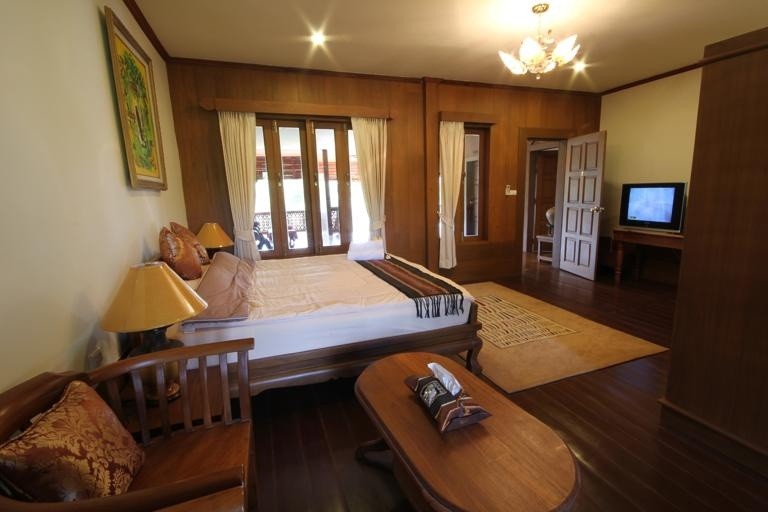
xmin=0 ymin=380 xmax=146 ymax=502
xmin=169 ymin=222 xmax=210 ymax=265
xmin=159 ymin=226 xmax=203 ymax=280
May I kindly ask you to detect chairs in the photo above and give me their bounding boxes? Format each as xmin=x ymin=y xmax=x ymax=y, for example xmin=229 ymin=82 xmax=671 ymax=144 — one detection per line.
xmin=1 ymin=338 xmax=255 ymax=512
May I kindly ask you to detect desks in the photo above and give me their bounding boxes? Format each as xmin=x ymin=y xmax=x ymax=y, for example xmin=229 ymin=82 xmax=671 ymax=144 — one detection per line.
xmin=354 ymin=351 xmax=581 ymax=512
xmin=536 ymin=235 xmax=554 ymax=262
xmin=613 ymin=226 xmax=685 ymax=287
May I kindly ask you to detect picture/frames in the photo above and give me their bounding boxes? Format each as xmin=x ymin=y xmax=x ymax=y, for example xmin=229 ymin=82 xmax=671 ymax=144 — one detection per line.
xmin=103 ymin=7 xmax=168 ymax=190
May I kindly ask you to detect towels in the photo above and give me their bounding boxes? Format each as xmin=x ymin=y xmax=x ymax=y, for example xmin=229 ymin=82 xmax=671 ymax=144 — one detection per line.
xmin=348 ymin=239 xmax=384 ymax=260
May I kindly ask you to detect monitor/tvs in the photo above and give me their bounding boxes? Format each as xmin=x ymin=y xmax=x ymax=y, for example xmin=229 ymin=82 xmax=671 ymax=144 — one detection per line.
xmin=619 ymin=182 xmax=687 ymax=234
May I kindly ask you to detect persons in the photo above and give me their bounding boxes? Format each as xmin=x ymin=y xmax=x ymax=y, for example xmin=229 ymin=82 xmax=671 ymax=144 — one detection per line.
xmin=252 ymin=220 xmax=274 ymax=250
xmin=286 ymin=224 xmax=297 ymax=248
xmin=267 ymin=226 xmax=273 ymax=242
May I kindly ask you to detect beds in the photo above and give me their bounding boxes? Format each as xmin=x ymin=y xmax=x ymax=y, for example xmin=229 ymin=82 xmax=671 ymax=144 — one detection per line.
xmin=127 ymin=253 xmax=483 ymax=397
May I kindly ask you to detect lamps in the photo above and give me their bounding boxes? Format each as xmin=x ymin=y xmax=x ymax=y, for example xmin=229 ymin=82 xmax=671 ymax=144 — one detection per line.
xmin=196 ymin=222 xmax=237 ymax=259
xmin=497 ymin=3 xmax=580 ymax=80
xmin=100 ymin=261 xmax=208 ymax=360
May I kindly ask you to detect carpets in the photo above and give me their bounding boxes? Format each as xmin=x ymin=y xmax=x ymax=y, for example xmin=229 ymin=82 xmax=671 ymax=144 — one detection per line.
xmin=457 ymin=280 xmax=670 ymax=393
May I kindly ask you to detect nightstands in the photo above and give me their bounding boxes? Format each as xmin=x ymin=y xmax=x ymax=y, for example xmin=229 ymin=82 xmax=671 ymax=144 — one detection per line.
xmin=125 ymin=365 xmax=223 ymax=433
